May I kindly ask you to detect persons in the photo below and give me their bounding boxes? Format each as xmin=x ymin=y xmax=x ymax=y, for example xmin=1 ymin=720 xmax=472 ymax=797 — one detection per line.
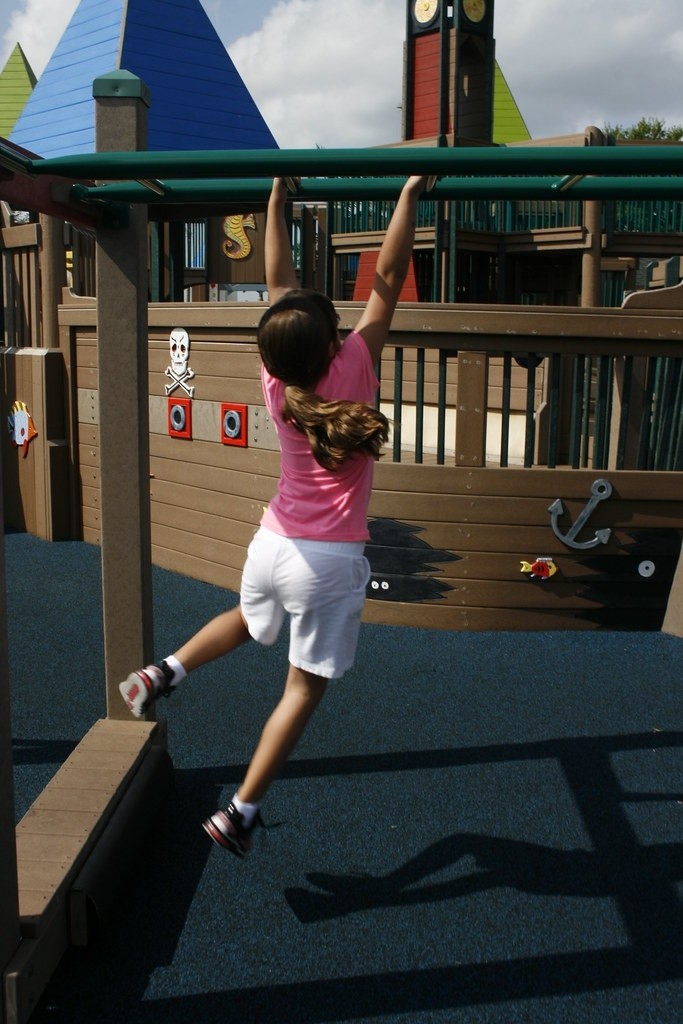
xmin=118 ymin=175 xmax=439 ymax=858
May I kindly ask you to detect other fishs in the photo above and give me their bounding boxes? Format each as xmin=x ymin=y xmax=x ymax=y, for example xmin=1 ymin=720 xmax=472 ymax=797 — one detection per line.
xmin=520 ymin=561 xmax=558 ymax=580
xmin=7 ymin=400 xmax=38 ymax=457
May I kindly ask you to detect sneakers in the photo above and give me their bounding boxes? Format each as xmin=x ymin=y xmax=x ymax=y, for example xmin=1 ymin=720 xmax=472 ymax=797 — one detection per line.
xmin=117 ymin=660 xmax=175 ymax=718
xmin=202 ymin=801 xmax=260 ymax=850
